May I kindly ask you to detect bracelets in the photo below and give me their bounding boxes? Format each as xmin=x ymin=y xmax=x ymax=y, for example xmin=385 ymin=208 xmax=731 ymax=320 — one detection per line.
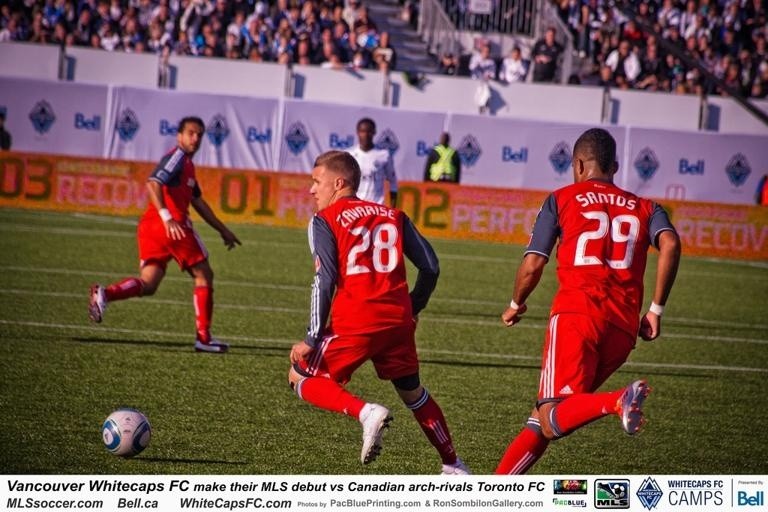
xmin=509 ymin=296 xmax=526 ymax=312
xmin=648 ymin=298 xmax=666 ymax=317
xmin=158 ymin=207 xmax=174 ymax=224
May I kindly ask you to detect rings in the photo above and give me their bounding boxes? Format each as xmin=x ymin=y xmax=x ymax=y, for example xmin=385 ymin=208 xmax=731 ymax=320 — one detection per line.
xmin=170 ymin=228 xmax=175 ymax=233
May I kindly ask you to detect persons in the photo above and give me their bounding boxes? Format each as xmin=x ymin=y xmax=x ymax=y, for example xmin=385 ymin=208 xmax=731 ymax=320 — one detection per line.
xmin=86 ymin=112 xmax=242 ymax=357
xmin=1 ymin=1 xmax=768 ymax=104
xmin=489 ymin=125 xmax=683 ymax=474
xmin=285 ymin=147 xmax=474 ymax=474
xmin=0 ymin=113 xmax=12 ymax=149
xmin=421 ymin=129 xmax=463 ymax=184
xmin=346 ymin=117 xmax=403 ymax=209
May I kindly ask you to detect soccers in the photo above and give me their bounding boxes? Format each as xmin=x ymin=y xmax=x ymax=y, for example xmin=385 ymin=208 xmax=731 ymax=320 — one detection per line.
xmin=102 ymin=408 xmax=151 ymax=457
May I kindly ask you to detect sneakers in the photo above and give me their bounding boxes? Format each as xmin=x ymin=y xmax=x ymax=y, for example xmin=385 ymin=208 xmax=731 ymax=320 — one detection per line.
xmin=617 ymin=380 xmax=652 ymax=435
xmin=195 ymin=339 xmax=230 ymax=354
xmin=89 ymin=283 xmax=105 ymax=324
xmin=359 ymin=403 xmax=393 ymax=466
xmin=441 ymin=458 xmax=471 ymax=475
